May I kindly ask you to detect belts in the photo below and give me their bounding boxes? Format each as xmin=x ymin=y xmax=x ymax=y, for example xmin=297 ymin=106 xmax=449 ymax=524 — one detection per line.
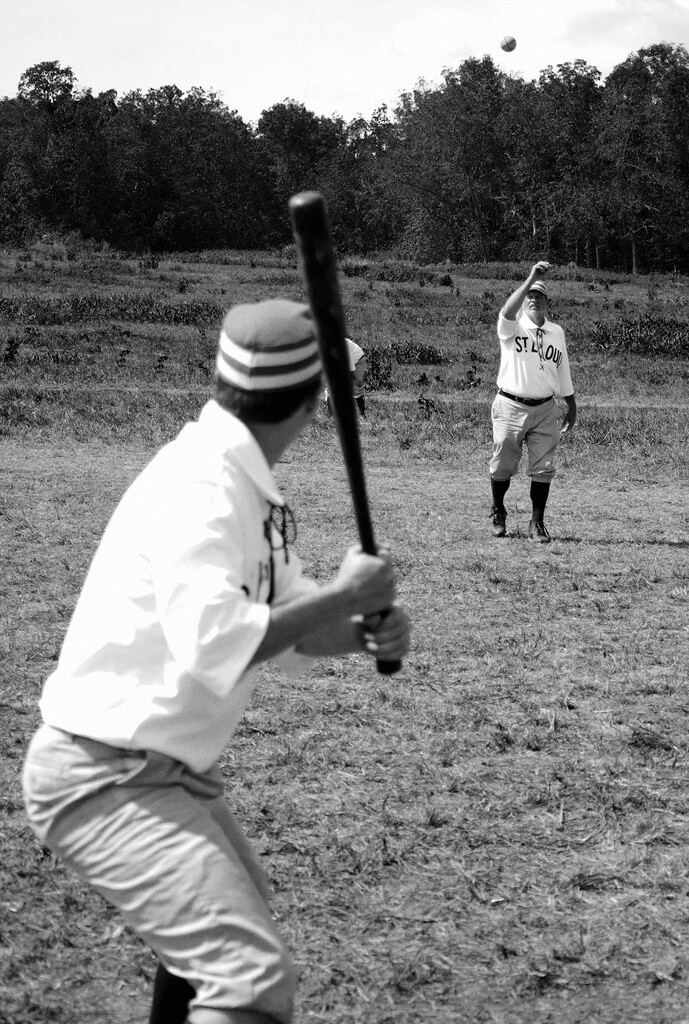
xmin=499 ymin=389 xmax=553 ymax=406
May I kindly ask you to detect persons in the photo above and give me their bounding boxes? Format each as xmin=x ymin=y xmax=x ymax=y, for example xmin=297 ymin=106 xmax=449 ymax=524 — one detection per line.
xmin=489 ymin=261 xmax=577 ymax=543
xmin=323 ymin=338 xmax=366 ymax=419
xmin=22 ymin=300 xmax=411 ymax=1024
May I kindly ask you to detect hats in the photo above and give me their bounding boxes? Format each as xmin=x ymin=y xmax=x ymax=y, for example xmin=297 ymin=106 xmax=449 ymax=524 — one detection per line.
xmin=529 ymin=281 xmax=549 ymax=299
xmin=217 ymin=299 xmax=323 ymax=392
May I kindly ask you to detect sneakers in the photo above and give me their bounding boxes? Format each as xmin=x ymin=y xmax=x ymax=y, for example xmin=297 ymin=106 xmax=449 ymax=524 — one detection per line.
xmin=488 ymin=504 xmax=508 ymax=537
xmin=528 ymin=521 xmax=552 ymax=543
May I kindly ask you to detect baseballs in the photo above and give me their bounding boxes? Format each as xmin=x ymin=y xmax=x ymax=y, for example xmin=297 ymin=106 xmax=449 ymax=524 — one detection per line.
xmin=500 ymin=36 xmax=516 ymax=52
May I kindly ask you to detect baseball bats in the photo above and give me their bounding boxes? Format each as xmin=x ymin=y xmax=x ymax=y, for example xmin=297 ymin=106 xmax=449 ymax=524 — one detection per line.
xmin=287 ymin=192 xmax=406 ymax=678
